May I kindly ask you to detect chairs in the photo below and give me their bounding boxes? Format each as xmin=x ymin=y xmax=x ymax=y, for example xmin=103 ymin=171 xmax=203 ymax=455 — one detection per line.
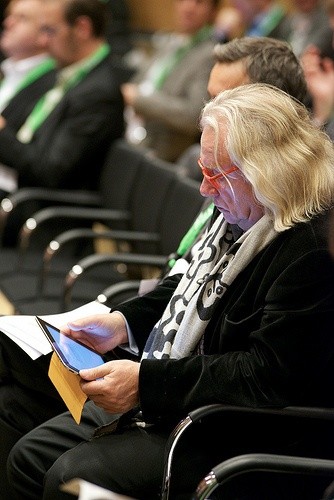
xmin=0 ymin=145 xmax=334 ymax=500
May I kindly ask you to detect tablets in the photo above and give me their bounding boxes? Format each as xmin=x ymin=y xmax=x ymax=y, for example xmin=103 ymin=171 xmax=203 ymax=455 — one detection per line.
xmin=36 ymin=316 xmax=106 ymax=381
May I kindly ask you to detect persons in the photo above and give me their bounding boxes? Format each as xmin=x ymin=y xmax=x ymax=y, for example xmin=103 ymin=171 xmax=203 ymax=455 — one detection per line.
xmin=0 ymin=0 xmax=334 ymax=463
xmin=5 ymin=82 xmax=334 ymax=500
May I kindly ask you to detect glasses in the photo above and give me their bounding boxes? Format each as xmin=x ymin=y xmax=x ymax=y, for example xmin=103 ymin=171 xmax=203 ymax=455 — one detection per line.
xmin=198 ymin=159 xmax=240 ymax=190
